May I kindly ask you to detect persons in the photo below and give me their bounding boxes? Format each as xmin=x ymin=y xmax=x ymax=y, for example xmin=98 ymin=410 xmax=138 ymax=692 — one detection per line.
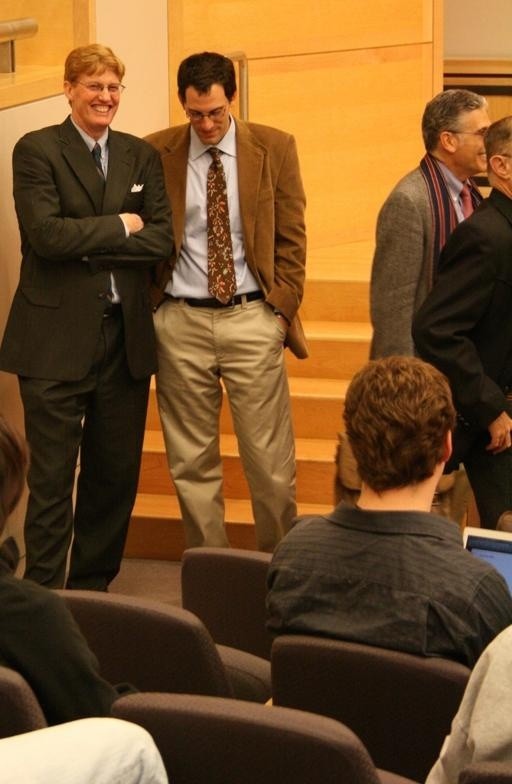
xmin=141 ymin=53 xmax=310 ymax=555
xmin=0 ymin=717 xmax=170 ymax=784
xmin=0 ymin=44 xmax=174 ymax=594
xmin=0 ymin=414 xmax=119 ymax=724
xmin=414 ymin=115 xmax=511 ymax=534
xmin=266 ymin=353 xmax=511 ymax=662
xmin=421 ymin=621 xmax=510 ymax=784
xmin=363 ymin=91 xmax=493 ymax=363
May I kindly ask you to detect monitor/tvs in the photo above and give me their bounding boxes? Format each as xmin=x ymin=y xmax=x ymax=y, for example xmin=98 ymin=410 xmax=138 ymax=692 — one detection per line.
xmin=462 ymin=526 xmax=512 ymax=600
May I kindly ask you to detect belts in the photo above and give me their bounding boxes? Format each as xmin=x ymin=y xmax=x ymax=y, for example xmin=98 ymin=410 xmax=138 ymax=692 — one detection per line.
xmin=102 ymin=304 xmax=123 ymax=317
xmin=160 ymin=289 xmax=266 ymax=309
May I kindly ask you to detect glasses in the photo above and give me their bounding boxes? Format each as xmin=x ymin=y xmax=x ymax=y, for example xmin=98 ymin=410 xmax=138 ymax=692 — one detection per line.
xmin=72 ymin=76 xmax=127 ymax=97
xmin=454 ymin=127 xmax=489 ymax=138
xmin=183 ymin=102 xmax=227 ymax=121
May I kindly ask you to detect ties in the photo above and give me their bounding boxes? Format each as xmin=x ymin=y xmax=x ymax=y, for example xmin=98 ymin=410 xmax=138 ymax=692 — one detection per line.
xmin=207 ymin=147 xmax=237 ymax=308
xmin=91 ymin=144 xmax=105 ymax=190
xmin=458 ymin=185 xmax=474 ymax=219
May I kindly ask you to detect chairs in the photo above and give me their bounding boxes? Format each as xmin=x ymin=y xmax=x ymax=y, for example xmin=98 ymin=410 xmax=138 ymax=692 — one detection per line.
xmin=50 ymin=586 xmax=272 ymax=706
xmin=0 ymin=663 xmax=47 ymax=738
xmin=180 ymin=547 xmax=280 ymax=655
xmin=112 ymin=693 xmax=407 ymax=784
xmin=273 ymin=631 xmax=472 ymax=781
